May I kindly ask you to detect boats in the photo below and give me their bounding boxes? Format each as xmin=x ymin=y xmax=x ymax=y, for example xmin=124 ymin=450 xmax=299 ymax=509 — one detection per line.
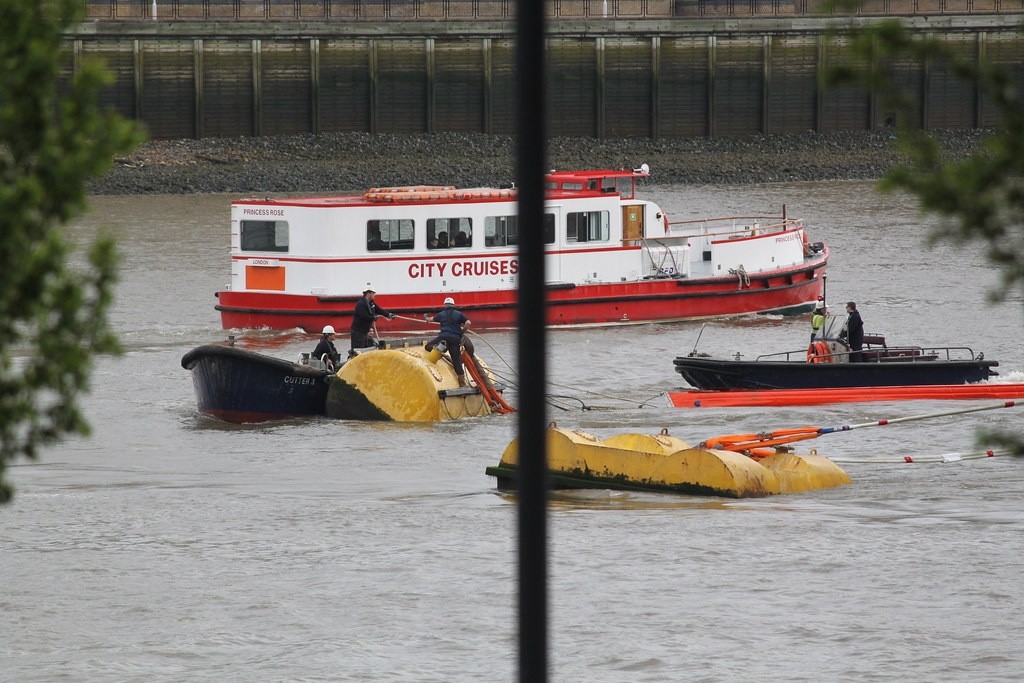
xmin=672 ymin=321 xmax=999 ymax=392
xmin=181 ymin=336 xmax=391 ymax=423
xmin=213 ymin=164 xmax=832 ymax=331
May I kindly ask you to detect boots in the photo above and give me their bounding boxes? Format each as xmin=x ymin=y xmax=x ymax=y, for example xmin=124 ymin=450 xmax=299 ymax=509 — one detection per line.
xmin=459 ymin=375 xmax=467 ymax=387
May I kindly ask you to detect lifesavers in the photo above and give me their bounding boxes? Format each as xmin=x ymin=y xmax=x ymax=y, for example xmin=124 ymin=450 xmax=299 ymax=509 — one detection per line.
xmin=661 ymin=209 xmax=669 ymax=233
xmin=802 ymin=230 xmax=808 ymax=256
xmin=807 ymin=340 xmax=832 ymax=364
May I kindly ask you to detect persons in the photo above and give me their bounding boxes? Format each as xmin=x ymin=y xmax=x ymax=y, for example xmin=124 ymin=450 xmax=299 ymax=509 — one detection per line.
xmin=424 ymin=296 xmax=471 ymax=389
xmin=368 ymin=230 xmax=389 ymax=251
xmin=428 ymin=230 xmax=471 ymax=249
xmin=312 ymin=324 xmax=341 ymax=373
xmin=810 ymin=300 xmax=864 ymax=362
xmin=350 ymin=287 xmax=395 ymax=349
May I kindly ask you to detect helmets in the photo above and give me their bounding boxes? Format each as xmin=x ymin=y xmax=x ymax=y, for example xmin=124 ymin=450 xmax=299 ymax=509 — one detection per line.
xmin=443 ymin=296 xmax=455 ymax=305
xmin=321 ymin=325 xmax=335 ymax=334
xmin=814 ymin=300 xmax=829 ymax=310
xmin=362 ymin=285 xmax=377 ymax=293
